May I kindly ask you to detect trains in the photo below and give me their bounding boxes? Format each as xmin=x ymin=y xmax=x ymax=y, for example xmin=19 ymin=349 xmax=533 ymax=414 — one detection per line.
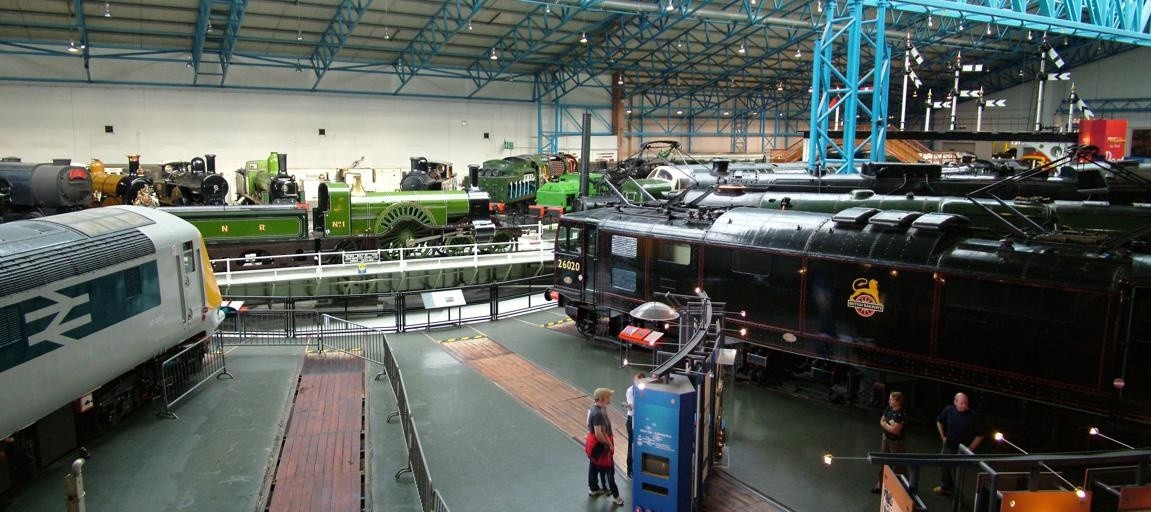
xmin=0 ymin=203 xmax=235 ymax=485
xmin=156 ymin=160 xmax=501 ymax=271
xmin=233 ymin=149 xmax=304 ymax=206
xmin=84 ymin=154 xmax=155 ymax=207
xmin=150 ymin=151 xmax=231 ymax=207
xmin=396 ymin=157 xmax=460 ymax=191
xmin=465 ymin=136 xmax=1151 ymax=268
xmin=0 ymin=155 xmax=95 ymax=224
xmin=537 ymin=139 xmax=1151 ymax=437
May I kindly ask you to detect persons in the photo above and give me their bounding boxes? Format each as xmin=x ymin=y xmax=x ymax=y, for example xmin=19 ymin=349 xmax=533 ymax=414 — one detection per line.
xmin=595 ymin=389 xmax=627 ymax=505
xmin=933 ymin=392 xmax=989 ymax=493
xmin=623 ymin=372 xmax=646 ymax=477
xmin=588 ymin=387 xmax=612 ymax=496
xmin=872 ymin=390 xmax=910 ymax=493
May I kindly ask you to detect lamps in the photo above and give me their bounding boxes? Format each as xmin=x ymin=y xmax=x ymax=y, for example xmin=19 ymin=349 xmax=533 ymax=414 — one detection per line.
xmin=104 ymin=0 xmax=112 ymax=18
xmin=676 ymin=102 xmax=683 ymax=114
xmin=490 ymin=47 xmax=498 ymax=60
xmin=295 ymin=44 xmax=302 ymax=72
xmin=794 ymin=48 xmax=801 ymax=57
xmin=580 ymin=9 xmax=587 ymax=43
xmin=208 ymin=19 xmax=213 ymax=31
xmin=384 ymin=0 xmax=390 ymax=39
xmin=618 ymin=74 xmax=623 ymax=84
xmin=65 ymin=0 xmax=79 ymax=51
xmin=626 ymin=104 xmax=632 ymax=113
xmin=186 ymin=24 xmax=193 ymax=71
xmin=296 ymin=0 xmax=304 ymax=41
xmin=738 ymin=39 xmax=745 ymax=53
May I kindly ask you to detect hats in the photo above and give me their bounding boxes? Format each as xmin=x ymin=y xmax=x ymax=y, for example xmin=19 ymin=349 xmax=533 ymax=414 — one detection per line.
xmin=593 ymin=388 xmax=615 ymax=401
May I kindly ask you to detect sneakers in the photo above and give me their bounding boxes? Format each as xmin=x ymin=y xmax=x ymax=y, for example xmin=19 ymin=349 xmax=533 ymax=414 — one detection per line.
xmin=932 ymin=486 xmax=953 ymax=494
xmin=612 ymin=494 xmax=624 ymax=505
xmin=588 ymin=488 xmax=605 ymax=496
